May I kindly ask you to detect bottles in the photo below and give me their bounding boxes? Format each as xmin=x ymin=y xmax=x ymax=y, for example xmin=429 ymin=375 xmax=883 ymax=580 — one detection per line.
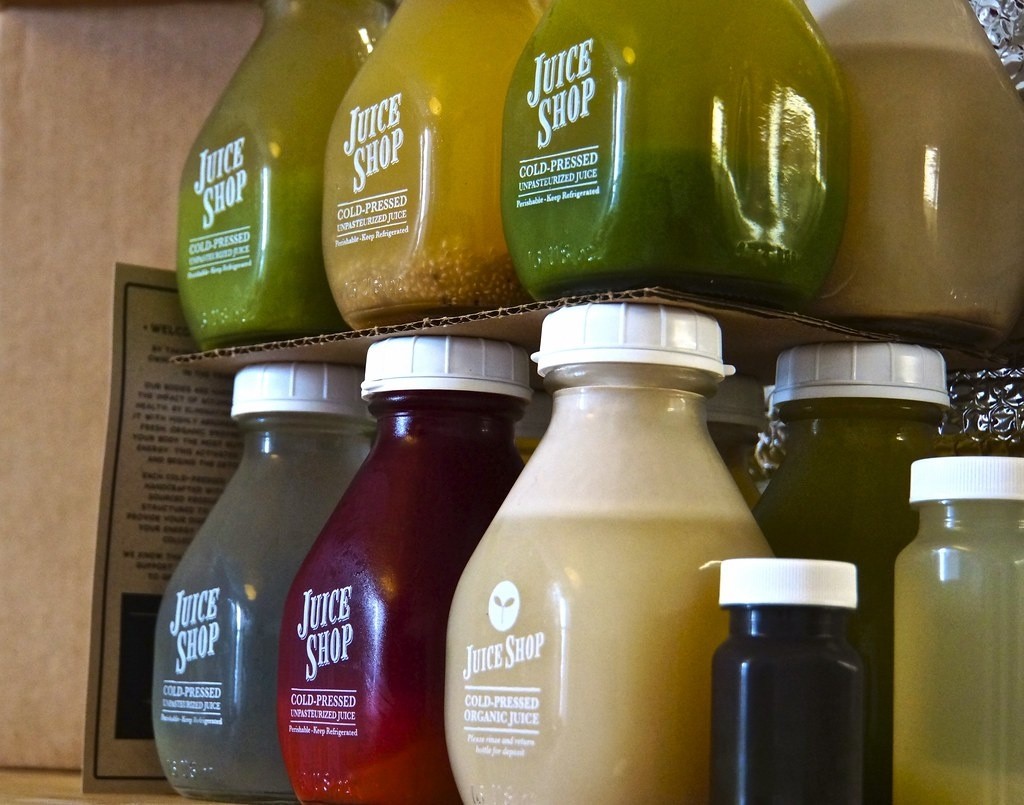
xmin=443 ymin=305 xmax=775 ymax=805
xmin=174 ymin=1 xmax=392 ymax=352
xmin=277 ymin=336 xmax=533 ymax=805
xmin=323 ymin=0 xmax=566 ymax=330
xmin=893 ymin=456 xmax=1024 ymax=805
xmin=801 ymin=0 xmax=1024 ymax=359
xmin=152 ymin=363 xmax=381 ymax=805
xmin=501 ymin=0 xmax=853 ymax=308
xmin=507 ymin=343 xmax=956 ymax=804
